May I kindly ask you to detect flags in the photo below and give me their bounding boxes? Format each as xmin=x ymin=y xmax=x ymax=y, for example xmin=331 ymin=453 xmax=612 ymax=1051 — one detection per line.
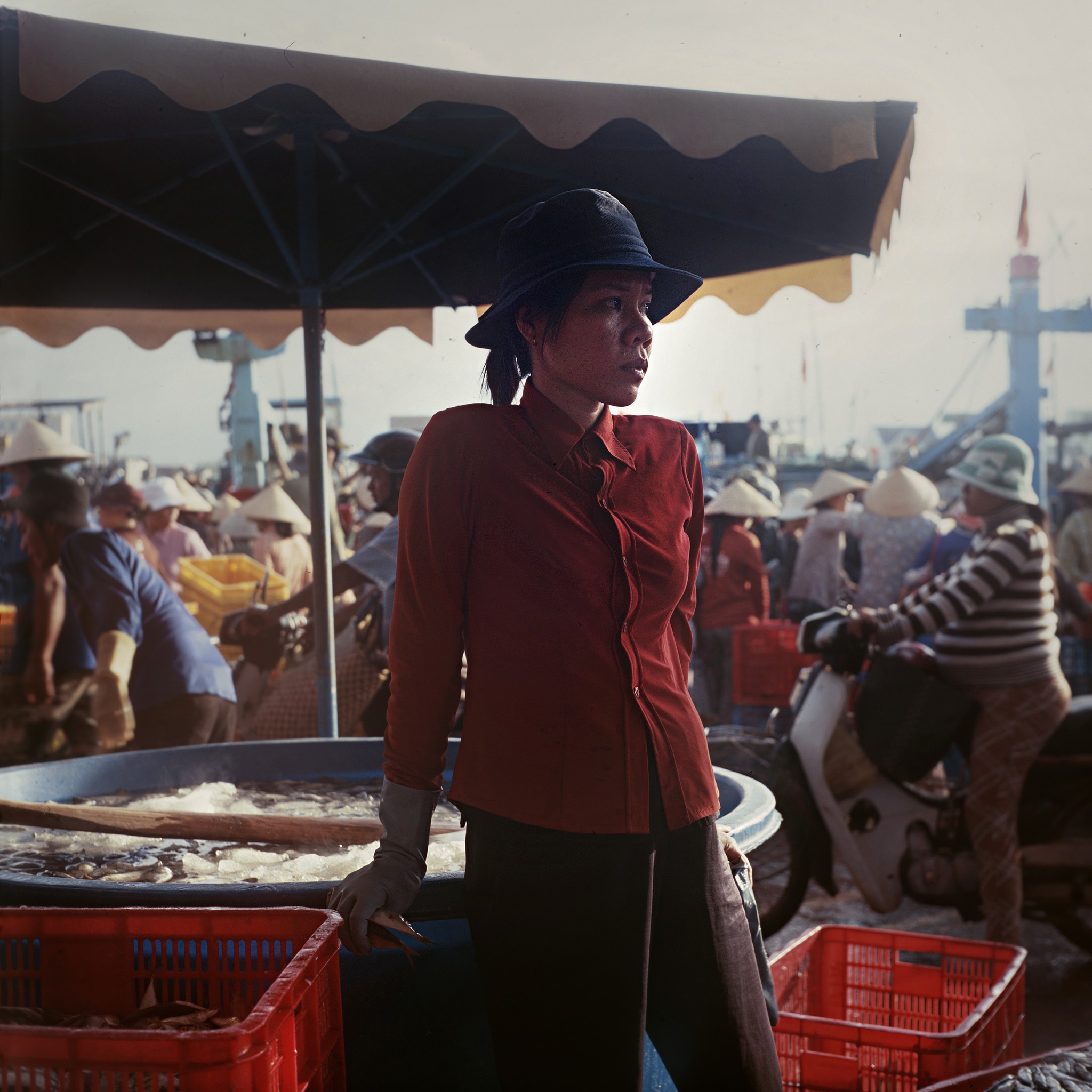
xmin=1015 ymin=191 xmax=1034 ymax=247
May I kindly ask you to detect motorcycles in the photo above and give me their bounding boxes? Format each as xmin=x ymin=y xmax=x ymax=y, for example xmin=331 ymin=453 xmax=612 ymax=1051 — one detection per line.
xmin=219 ymin=562 xmax=1090 ymax=955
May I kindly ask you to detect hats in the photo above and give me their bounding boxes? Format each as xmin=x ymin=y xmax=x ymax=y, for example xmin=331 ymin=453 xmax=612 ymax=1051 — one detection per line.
xmin=863 ymin=466 xmax=940 ymax=518
xmin=1058 ymin=467 xmax=1092 ymax=495
xmin=174 ymin=474 xmax=212 ymax=512
xmin=142 ymin=476 xmax=188 ymax=510
xmin=218 ymin=508 xmax=261 ymax=541
xmin=805 ymin=468 xmax=869 ymax=510
xmin=290 ymin=517 xmax=311 ymax=534
xmin=945 ymin=435 xmax=1040 ymax=506
xmin=232 ymin=483 xmax=307 ymax=525
xmin=464 ymin=188 xmax=704 ymax=351
xmin=704 ymin=478 xmax=781 ymax=518
xmin=775 ymin=488 xmax=818 ymax=521
xmin=206 ymin=492 xmax=242 ymax=524
xmin=0 ymin=417 xmax=91 ymax=466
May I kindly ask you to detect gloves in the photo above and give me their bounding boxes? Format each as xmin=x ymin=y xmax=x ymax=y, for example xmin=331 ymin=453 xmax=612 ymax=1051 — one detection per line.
xmin=328 ymin=775 xmax=443 ymax=953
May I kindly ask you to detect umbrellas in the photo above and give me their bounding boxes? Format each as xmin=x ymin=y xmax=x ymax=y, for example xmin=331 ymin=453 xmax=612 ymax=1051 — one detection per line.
xmin=0 ymin=7 xmax=915 ymax=737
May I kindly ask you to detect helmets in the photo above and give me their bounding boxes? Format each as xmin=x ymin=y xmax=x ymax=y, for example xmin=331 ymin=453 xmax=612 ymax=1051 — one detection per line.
xmin=351 ymin=429 xmax=421 ymax=475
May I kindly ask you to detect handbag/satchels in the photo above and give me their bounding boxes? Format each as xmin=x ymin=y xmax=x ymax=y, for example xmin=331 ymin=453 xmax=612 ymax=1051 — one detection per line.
xmin=854 ymin=648 xmax=983 ymax=784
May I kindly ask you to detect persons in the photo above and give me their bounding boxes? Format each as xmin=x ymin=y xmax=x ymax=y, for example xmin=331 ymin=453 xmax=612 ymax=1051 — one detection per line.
xmin=329 ymin=189 xmax=789 ymax=1092
xmin=0 ymin=409 xmax=1092 ymax=969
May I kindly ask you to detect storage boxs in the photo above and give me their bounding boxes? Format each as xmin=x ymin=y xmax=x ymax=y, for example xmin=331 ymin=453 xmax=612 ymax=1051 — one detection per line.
xmin=768 ymin=922 xmax=1028 ymax=1092
xmin=170 ymin=554 xmax=293 ymax=665
xmin=0 ymin=906 xmax=351 ymax=1092
xmin=730 ymin=620 xmax=871 ymax=710
